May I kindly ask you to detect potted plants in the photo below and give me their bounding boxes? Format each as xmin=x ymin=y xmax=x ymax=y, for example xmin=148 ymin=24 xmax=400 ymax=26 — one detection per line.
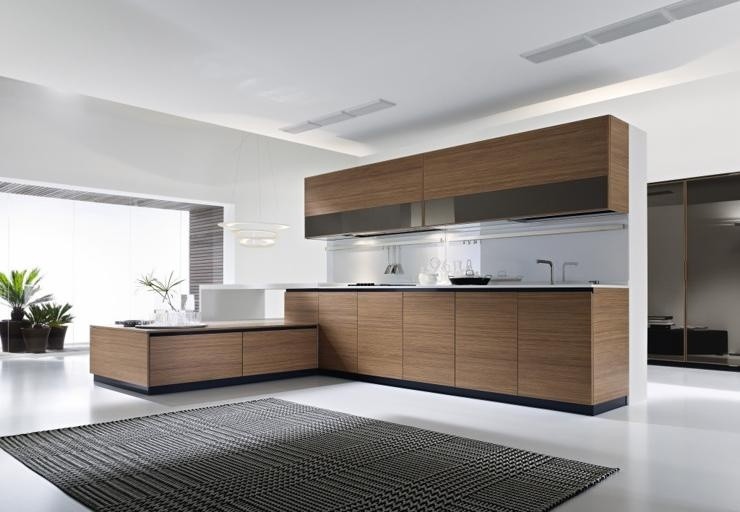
xmin=0 ymin=269 xmax=75 ymax=353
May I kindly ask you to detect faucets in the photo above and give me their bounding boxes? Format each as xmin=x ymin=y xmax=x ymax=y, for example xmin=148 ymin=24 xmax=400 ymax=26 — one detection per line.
xmin=536 ymin=258 xmax=555 ymax=282
xmin=562 ymin=261 xmax=578 ymax=281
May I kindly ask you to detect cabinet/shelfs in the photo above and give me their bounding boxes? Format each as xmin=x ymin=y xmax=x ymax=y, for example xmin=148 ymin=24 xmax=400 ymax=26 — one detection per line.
xmin=454 ymin=290 xmax=596 ymax=404
xmin=283 ymin=292 xmax=355 ymax=373
xmin=355 ymin=292 xmax=456 ymax=388
xmin=423 ymin=115 xmax=611 ymax=226
xmin=92 ymin=323 xmax=315 ymax=392
xmin=305 ymin=151 xmax=424 ymax=238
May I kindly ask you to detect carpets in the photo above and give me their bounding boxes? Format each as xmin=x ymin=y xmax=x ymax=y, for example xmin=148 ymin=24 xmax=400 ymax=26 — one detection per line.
xmin=0 ymin=397 xmax=620 ymax=512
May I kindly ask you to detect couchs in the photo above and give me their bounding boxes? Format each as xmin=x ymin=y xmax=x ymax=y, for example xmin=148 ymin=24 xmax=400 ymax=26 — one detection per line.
xmin=648 ymin=329 xmax=728 ymax=355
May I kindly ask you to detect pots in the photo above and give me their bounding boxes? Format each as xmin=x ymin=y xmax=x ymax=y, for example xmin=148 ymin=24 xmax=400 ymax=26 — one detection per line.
xmin=448 ymin=274 xmax=492 ymax=285
